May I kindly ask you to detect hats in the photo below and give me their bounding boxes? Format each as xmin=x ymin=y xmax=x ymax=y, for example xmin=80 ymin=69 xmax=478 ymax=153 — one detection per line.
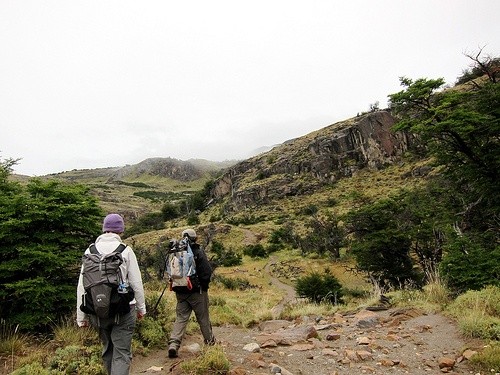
xmin=181 ymin=229 xmax=197 ymax=240
xmin=103 ymin=214 xmax=124 ymax=233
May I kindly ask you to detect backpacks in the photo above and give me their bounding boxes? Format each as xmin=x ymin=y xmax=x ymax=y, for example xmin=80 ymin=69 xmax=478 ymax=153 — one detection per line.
xmin=163 ymin=236 xmax=196 ymax=293
xmin=81 ymin=242 xmax=134 ymax=327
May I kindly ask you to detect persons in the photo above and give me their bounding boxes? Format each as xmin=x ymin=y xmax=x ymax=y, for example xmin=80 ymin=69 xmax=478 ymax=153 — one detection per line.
xmin=76 ymin=214 xmax=146 ymax=375
xmin=168 ymin=229 xmax=215 ymax=358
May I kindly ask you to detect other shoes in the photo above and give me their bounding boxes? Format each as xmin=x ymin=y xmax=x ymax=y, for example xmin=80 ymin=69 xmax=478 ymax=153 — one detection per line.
xmin=168 ymin=343 xmax=180 ymax=358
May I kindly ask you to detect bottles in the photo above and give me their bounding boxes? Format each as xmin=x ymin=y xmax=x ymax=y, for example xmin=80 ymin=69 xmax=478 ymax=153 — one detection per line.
xmin=118 ymin=278 xmax=129 ymax=293
